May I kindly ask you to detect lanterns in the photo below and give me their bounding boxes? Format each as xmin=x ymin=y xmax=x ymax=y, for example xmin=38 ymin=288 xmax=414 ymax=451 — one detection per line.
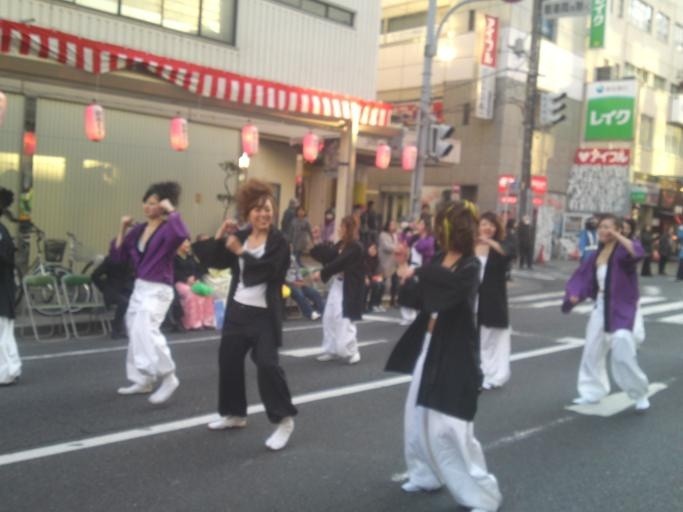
xmin=375 ymin=142 xmax=391 ymax=169
xmin=241 ymin=119 xmax=259 ymax=156
xmin=85 ymin=98 xmax=106 ymax=142
xmin=0 ymin=91 xmax=7 ymax=126
xmin=401 ymin=143 xmax=418 ymax=172
xmin=301 ymin=129 xmax=321 ymax=163
xmin=170 ymin=112 xmax=189 ymax=152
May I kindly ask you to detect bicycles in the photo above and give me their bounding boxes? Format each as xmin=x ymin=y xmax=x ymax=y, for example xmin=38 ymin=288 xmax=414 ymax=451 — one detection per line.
xmin=4 ymin=208 xmax=107 ymax=316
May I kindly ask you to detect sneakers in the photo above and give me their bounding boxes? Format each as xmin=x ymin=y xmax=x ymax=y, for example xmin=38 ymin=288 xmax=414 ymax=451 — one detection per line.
xmin=573 ymin=396 xmax=599 ymax=405
xmin=481 ymin=381 xmax=501 ymax=390
xmin=402 ymin=481 xmax=423 ymax=491
xmin=118 ymin=384 xmax=153 ymax=395
xmin=266 ymin=419 xmax=295 ymax=450
xmin=209 ymin=417 xmax=248 ymax=428
xmin=350 ymin=352 xmax=361 ymax=364
xmin=470 ymin=507 xmax=490 ymax=512
xmin=316 ymin=353 xmax=341 ymax=361
xmin=309 ymin=311 xmax=322 ymax=322
xmin=635 ymin=396 xmax=651 ymax=411
xmin=148 ymin=377 xmax=179 ymax=404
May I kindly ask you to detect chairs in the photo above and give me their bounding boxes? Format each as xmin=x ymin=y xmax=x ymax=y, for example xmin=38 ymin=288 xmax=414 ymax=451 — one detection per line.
xmin=60 ymin=276 xmax=106 ymax=340
xmin=21 ymin=274 xmax=69 ymax=343
xmin=285 ymin=268 xmax=327 ymax=318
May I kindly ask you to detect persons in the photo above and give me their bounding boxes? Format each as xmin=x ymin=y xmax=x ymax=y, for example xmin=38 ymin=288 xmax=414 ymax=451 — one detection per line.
xmin=382 ymin=199 xmax=500 ymax=512
xmin=562 ymin=214 xmax=649 ymax=409
xmin=196 ymin=181 xmax=298 ymax=449
xmin=0 ymin=184 xmax=22 ymax=386
xmin=109 ymin=180 xmax=192 ymax=404
xmin=88 ymin=198 xmax=444 ymax=336
xmin=473 ymin=211 xmax=511 ymax=390
xmin=313 ymin=216 xmax=363 ymax=364
xmin=579 ymin=217 xmax=683 ymax=279
xmin=505 ymin=213 xmax=533 ymax=270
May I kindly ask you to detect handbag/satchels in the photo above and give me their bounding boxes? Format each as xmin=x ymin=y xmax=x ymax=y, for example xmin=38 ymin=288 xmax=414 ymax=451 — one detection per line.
xmin=190 ymin=282 xmax=213 ymax=295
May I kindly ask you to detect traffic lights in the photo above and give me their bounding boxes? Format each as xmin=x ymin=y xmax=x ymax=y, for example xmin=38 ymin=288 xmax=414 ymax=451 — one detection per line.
xmin=429 ymin=123 xmax=453 ymax=158
xmin=537 ymin=90 xmax=567 ymax=128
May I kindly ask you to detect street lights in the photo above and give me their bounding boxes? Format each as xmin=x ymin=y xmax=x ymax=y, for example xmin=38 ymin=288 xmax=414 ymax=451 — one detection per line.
xmin=408 ymin=0 xmax=522 ymax=225
